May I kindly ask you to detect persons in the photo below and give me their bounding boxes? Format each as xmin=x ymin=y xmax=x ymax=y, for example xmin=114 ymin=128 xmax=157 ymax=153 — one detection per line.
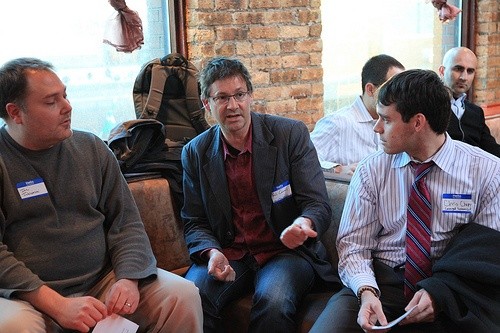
xmin=308 ymin=69 xmax=500 ymax=333
xmin=310 ymin=54 xmax=407 ymax=175
xmin=0 ymin=58 xmax=203 ymax=333
xmin=181 ymin=57 xmax=342 ymax=333
xmin=439 ymin=47 xmax=500 ymax=158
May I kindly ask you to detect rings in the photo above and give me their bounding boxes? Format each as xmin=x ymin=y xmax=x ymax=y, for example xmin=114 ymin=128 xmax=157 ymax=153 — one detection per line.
xmin=125 ymin=302 xmax=131 ymax=307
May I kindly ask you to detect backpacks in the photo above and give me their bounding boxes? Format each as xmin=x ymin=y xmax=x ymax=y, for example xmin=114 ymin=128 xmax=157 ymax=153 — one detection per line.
xmin=133 ymin=53 xmax=211 ymax=152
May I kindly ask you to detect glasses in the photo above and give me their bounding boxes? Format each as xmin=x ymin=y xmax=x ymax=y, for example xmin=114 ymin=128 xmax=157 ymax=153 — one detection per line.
xmin=205 ymin=90 xmax=250 ymax=105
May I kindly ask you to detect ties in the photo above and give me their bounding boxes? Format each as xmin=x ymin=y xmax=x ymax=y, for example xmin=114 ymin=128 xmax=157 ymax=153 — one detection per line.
xmin=403 ymin=160 xmax=436 ymax=301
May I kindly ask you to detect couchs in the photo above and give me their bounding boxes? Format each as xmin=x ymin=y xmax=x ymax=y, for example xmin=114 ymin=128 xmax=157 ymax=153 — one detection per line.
xmin=123 ymin=171 xmax=349 ymax=333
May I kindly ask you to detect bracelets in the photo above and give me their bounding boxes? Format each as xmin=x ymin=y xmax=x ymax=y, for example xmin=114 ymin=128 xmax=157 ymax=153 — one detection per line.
xmin=358 ymin=288 xmax=379 ymax=308
